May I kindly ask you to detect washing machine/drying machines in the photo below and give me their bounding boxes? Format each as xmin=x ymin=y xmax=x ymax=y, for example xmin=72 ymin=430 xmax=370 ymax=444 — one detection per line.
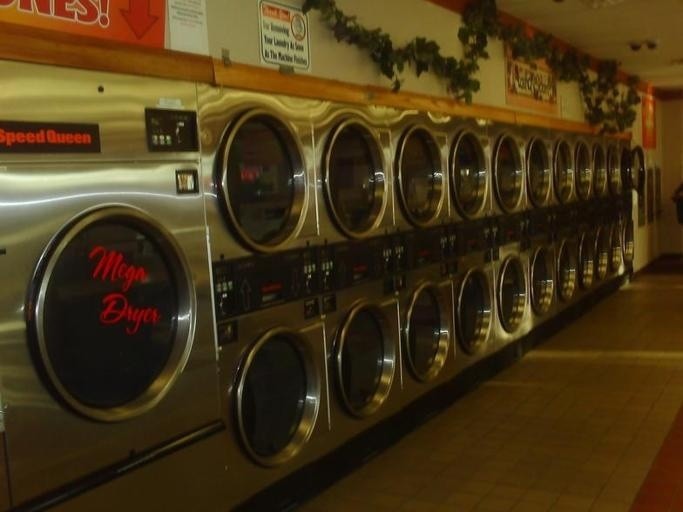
xmin=1 ymin=21 xmax=645 ymax=512
